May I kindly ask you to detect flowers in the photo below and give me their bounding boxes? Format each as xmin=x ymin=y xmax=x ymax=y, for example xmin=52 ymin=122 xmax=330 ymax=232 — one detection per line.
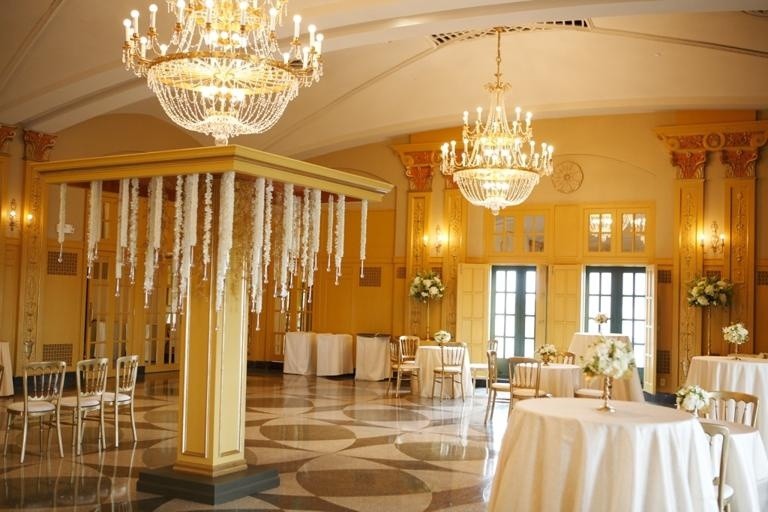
xmin=593 ymin=311 xmax=609 ymax=322
xmin=721 ymin=320 xmax=751 ymax=344
xmin=407 ymin=268 xmax=445 ymax=302
xmin=686 ymin=277 xmax=735 ymax=313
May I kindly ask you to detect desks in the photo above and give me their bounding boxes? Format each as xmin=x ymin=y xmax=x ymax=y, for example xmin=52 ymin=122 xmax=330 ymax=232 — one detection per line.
xmin=354 ymin=331 xmax=392 ymax=381
xmin=282 ymin=331 xmax=316 ymax=376
xmin=92 ymin=319 xmax=174 ymax=363
xmin=314 ymin=333 xmax=353 ymax=379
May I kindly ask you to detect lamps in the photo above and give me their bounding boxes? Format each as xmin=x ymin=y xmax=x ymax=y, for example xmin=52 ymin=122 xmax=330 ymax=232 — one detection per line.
xmin=119 ymin=1 xmax=325 ymax=148
xmin=435 ymin=28 xmax=556 ymax=218
xmin=421 ymin=222 xmax=449 ymax=252
xmin=8 ymin=198 xmax=17 ymax=231
xmin=698 ymin=222 xmax=725 ymax=254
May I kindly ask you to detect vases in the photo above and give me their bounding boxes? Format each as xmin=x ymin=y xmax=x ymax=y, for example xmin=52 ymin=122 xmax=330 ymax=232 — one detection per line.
xmin=598 ymin=322 xmax=603 ymax=332
xmin=704 ymin=304 xmax=712 ymax=355
xmin=423 ymin=301 xmax=432 ymax=341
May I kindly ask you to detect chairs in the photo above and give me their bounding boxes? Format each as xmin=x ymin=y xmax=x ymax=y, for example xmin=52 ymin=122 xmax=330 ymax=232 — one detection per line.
xmin=2 ymin=358 xmax=67 ymax=464
xmin=53 ymin=445 xmax=108 ymax=512
xmin=389 ymin=332 xmax=768 ymax=512
xmin=99 ymin=441 xmax=137 ymax=512
xmin=2 ymin=453 xmax=65 ymax=512
xmin=97 ymin=352 xmax=140 ymax=446
xmin=45 ymin=356 xmax=108 ymax=455
